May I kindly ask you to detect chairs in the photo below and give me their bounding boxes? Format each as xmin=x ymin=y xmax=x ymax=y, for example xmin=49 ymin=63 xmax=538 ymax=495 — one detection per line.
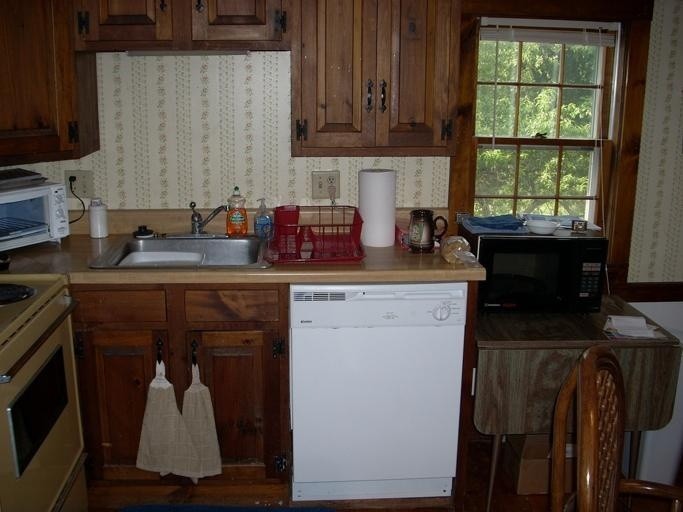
xmin=549 ymin=345 xmax=683 ymax=512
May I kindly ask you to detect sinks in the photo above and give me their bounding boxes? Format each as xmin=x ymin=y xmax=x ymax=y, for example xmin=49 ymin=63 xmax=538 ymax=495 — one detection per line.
xmin=90 ymin=232 xmax=274 ymax=270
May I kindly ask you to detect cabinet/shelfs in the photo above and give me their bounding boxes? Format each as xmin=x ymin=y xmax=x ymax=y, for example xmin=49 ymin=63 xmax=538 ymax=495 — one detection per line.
xmin=0 ymin=0 xmax=100 ymax=166
xmin=289 ymin=0 xmax=456 ymax=157
xmin=69 ymin=283 xmax=289 ymax=481
xmin=69 ymin=0 xmax=291 ymax=52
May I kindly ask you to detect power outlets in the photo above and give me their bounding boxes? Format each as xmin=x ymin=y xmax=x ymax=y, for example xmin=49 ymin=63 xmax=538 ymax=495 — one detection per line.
xmin=312 ymin=171 xmax=340 ymax=199
xmin=64 ymin=170 xmax=94 ymax=198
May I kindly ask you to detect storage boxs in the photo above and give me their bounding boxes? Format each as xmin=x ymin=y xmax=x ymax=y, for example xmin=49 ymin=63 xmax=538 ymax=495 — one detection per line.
xmin=517 ymin=434 xmax=576 ymax=495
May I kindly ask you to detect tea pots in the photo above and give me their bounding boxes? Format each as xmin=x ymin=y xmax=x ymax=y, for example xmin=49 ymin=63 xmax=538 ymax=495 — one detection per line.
xmin=408 ymin=209 xmax=448 ymax=254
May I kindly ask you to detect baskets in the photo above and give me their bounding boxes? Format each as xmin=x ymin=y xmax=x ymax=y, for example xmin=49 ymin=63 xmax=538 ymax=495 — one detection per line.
xmin=274 ymin=205 xmax=364 ymax=258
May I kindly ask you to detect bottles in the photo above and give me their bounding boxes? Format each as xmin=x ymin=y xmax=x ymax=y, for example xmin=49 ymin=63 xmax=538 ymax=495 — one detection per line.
xmin=296 ymin=226 xmax=317 ymax=260
xmin=90 ymin=237 xmax=108 ymax=259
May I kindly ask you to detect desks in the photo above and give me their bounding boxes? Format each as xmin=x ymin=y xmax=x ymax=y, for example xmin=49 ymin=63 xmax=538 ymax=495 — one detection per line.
xmin=473 ymin=292 xmax=682 ymax=512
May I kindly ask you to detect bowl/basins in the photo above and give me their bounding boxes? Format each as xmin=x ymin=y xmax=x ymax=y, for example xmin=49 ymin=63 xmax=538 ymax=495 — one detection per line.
xmin=524 ymin=219 xmax=560 ymax=235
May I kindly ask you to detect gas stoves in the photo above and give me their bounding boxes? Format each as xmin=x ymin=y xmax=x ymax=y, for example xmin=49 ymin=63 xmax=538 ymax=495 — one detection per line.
xmin=0 ymin=272 xmax=69 ymax=349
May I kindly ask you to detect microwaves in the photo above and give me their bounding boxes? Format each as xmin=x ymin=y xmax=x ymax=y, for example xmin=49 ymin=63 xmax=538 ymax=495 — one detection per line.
xmin=1 ymin=178 xmax=69 ymax=256
xmin=458 ymin=216 xmax=609 ymax=315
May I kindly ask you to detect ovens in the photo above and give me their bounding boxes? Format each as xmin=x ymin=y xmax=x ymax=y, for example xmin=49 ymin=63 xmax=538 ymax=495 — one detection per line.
xmin=0 ymin=285 xmax=89 ymax=512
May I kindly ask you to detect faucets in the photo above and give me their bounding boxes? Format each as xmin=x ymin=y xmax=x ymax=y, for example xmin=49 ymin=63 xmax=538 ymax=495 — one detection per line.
xmin=201 ymin=204 xmax=229 ymax=228
